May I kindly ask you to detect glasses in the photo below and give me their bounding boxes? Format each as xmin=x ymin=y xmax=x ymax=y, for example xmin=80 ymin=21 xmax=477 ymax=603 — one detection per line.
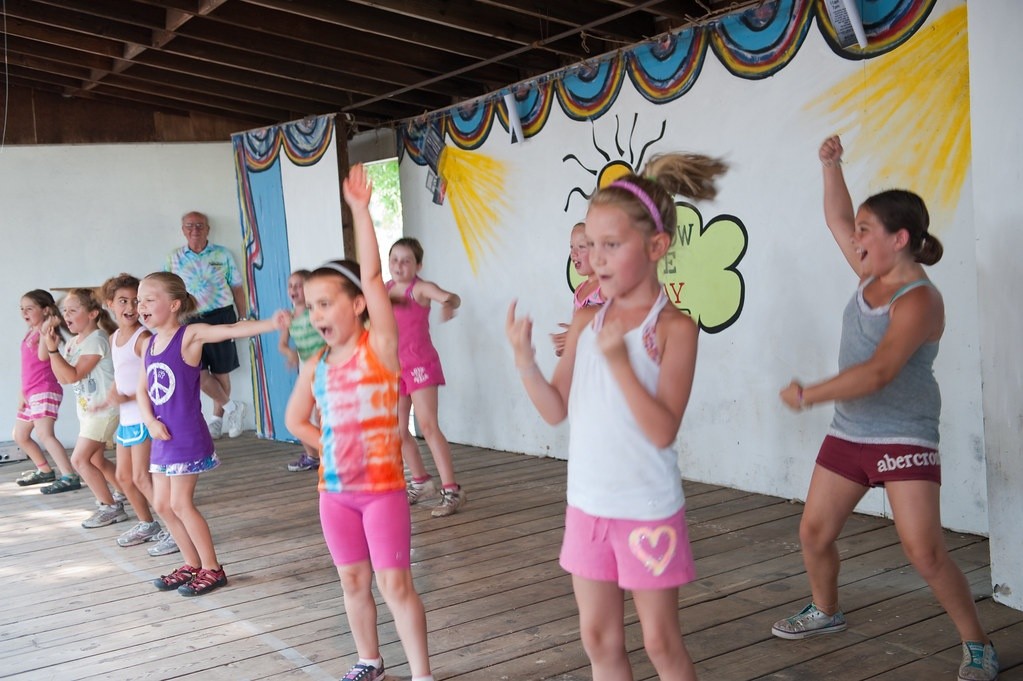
xmin=182 ymin=223 xmax=206 ymax=229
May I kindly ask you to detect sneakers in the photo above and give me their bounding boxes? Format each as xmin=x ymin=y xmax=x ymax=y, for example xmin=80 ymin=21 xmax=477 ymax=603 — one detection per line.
xmin=81 ymin=500 xmax=128 ymax=527
xmin=112 ymin=489 xmax=125 ymax=503
xmin=228 ymin=400 xmax=247 ymax=437
xmin=117 ymin=521 xmax=161 ymax=553
xmin=337 ymin=656 xmax=385 ymax=681
xmin=287 ymin=453 xmax=320 ymax=471
xmin=771 ymin=603 xmax=847 ymax=637
xmin=958 ymin=638 xmax=999 ymax=681
xmin=206 ymin=415 xmax=223 ymax=439
xmin=406 ymin=475 xmax=434 ymax=502
xmin=432 ymin=484 xmax=466 ymax=517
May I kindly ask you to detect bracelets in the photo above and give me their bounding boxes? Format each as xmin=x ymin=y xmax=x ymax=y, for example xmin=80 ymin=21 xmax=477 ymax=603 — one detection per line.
xmin=46 ymin=349 xmax=59 ymax=353
xmin=796 ymin=384 xmax=813 ymax=411
xmin=445 ymin=300 xmax=452 ymax=306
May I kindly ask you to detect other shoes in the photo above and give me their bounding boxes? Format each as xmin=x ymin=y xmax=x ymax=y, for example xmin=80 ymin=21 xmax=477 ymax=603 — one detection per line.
xmin=148 ymin=530 xmax=178 ymax=556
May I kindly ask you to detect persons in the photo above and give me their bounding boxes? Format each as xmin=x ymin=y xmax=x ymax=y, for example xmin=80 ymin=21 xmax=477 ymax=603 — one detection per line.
xmin=37 ymin=289 xmax=131 ymax=528
xmin=281 ymin=161 xmax=442 ymax=681
xmin=13 ymin=289 xmax=82 ymax=496
xmin=771 ymin=134 xmax=1000 ymax=681
xmin=83 ymin=274 xmax=181 ymax=556
xmin=132 ymin=272 xmax=294 ymax=596
xmin=276 ymin=268 xmax=331 ymax=473
xmin=552 ymin=220 xmax=607 ymax=357
xmin=162 ymin=212 xmax=248 ymax=441
xmin=500 ymin=149 xmax=730 ymax=681
xmin=383 ymin=234 xmax=465 ymax=517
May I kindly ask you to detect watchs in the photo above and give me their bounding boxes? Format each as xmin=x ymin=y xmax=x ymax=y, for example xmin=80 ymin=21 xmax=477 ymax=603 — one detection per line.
xmin=238 ymin=317 xmax=247 ymax=321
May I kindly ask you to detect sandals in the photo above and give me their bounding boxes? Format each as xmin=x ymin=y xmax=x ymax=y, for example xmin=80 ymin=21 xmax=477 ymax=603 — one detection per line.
xmin=154 ymin=563 xmax=202 ymax=590
xmin=177 ymin=564 xmax=228 ymax=595
xmin=16 ymin=469 xmax=54 ymax=485
xmin=40 ymin=475 xmax=80 ymax=494
